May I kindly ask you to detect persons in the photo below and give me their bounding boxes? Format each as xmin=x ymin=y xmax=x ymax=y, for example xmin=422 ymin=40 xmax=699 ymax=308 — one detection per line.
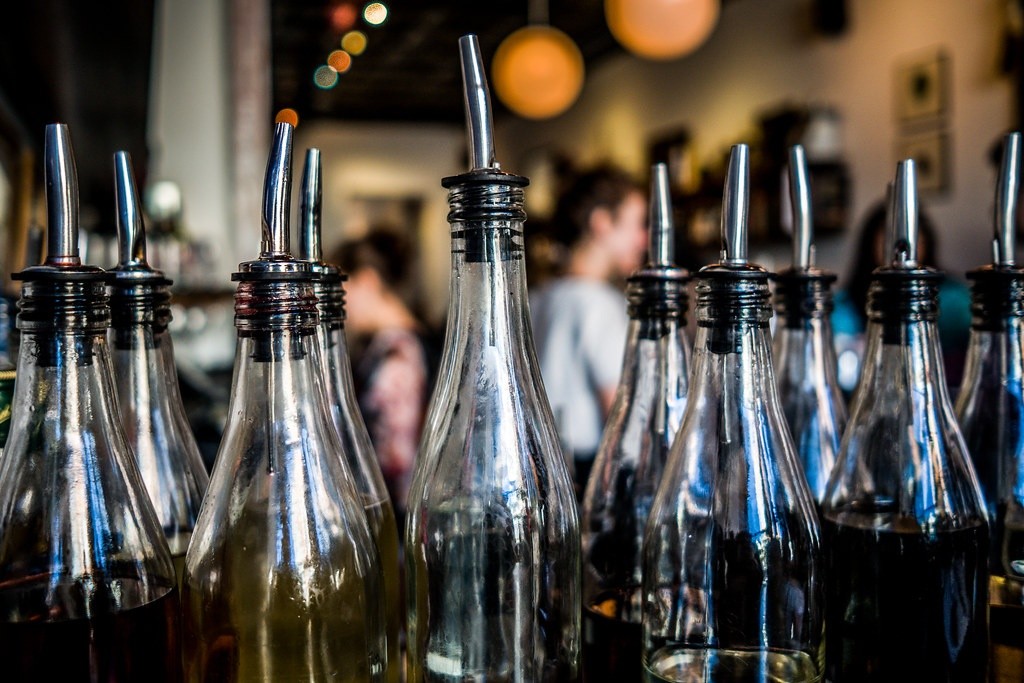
xmin=524 ymin=160 xmax=649 ymax=504
xmin=827 ymin=199 xmax=970 ymax=414
xmin=328 ymin=223 xmax=434 ymax=541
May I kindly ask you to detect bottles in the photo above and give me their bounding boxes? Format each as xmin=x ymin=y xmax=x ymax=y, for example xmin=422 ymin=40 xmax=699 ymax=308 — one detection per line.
xmin=0 ymin=36 xmax=1023 ymax=682
